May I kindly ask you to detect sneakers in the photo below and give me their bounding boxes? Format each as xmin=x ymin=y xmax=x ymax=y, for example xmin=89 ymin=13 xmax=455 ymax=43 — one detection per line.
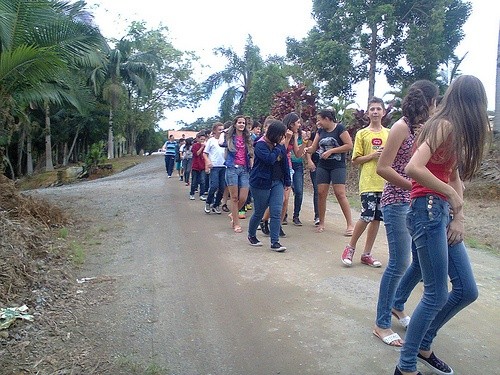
xmin=205 ymin=203 xmax=211 ymax=213
xmin=247 ymin=235 xmax=262 ymax=246
xmin=271 ymin=242 xmax=286 ymax=251
xmin=341 ymin=244 xmax=355 ymax=266
xmin=259 ymin=218 xmax=270 ymax=236
xmin=279 ymin=226 xmax=286 ymax=237
xmin=361 ymin=254 xmax=382 ymax=267
xmin=293 ymin=218 xmax=303 ymax=226
xmin=212 ymin=206 xmax=221 ymax=214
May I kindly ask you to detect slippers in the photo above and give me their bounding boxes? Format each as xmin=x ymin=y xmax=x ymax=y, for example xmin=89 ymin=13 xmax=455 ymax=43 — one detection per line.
xmin=391 ymin=311 xmax=410 ymax=328
xmin=373 ymin=330 xmax=404 ymax=347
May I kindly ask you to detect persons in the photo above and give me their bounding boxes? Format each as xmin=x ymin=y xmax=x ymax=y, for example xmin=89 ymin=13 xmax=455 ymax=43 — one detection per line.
xmin=373 ymin=79 xmax=440 ymax=347
xmin=301 ymin=108 xmax=354 ymax=236
xmin=341 ymin=95 xmax=390 ymax=267
xmin=162 ymin=113 xmax=320 ymax=238
xmin=246 ymin=121 xmax=291 ymax=251
xmin=393 ymin=76 xmax=492 ymax=375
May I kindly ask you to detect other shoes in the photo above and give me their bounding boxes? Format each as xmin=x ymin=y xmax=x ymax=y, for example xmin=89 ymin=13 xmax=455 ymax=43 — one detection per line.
xmin=239 ymin=210 xmax=246 ymax=219
xmin=233 ymin=222 xmax=242 ymax=233
xmin=345 ymin=227 xmax=354 ymax=236
xmin=314 ymin=218 xmax=319 ymax=225
xmin=222 ymin=204 xmax=229 ymax=212
xmin=167 ymin=175 xmax=206 ymax=201
xmin=282 ymin=220 xmax=287 ymax=225
xmin=314 ymin=226 xmax=324 ymax=233
xmin=417 ymin=350 xmax=453 ymax=375
xmin=246 ymin=204 xmax=253 ymax=210
xmin=228 ymin=213 xmax=233 ymax=220
xmin=394 ymin=366 xmax=423 ymax=375
